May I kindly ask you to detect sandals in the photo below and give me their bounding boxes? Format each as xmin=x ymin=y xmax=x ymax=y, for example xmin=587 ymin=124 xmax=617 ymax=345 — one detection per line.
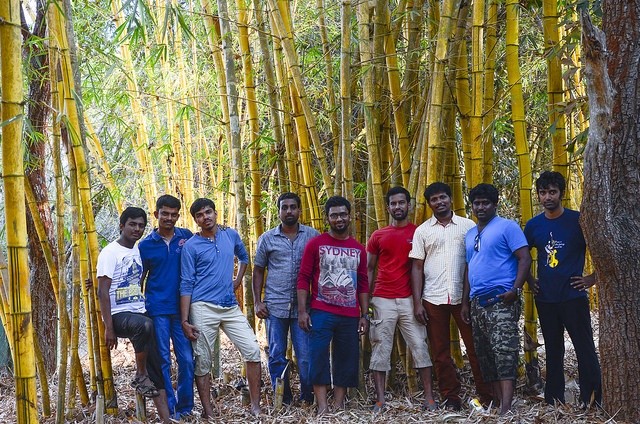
xmin=129 ymin=370 xmax=161 ymax=397
xmin=370 ymin=401 xmax=388 ymax=417
xmin=423 ymin=397 xmax=437 ymax=413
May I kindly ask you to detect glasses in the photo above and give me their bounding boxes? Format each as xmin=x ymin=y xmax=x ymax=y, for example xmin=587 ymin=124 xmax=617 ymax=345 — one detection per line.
xmin=474 ymin=234 xmax=480 ymax=252
xmin=328 ymin=212 xmax=350 ymax=219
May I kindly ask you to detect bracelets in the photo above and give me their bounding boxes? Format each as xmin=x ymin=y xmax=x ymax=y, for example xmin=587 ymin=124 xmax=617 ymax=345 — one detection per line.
xmin=182 ymin=320 xmax=188 ymax=326
xmin=361 ymin=313 xmax=369 ymax=320
xmin=511 ymin=286 xmax=521 ymax=295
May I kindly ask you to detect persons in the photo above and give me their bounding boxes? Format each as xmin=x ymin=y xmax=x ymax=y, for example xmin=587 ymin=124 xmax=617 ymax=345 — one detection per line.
xmin=179 ymin=197 xmax=261 ymax=420
xmin=367 ymin=187 xmax=435 ymax=414
xmin=524 ymin=172 xmax=602 ymax=413
xmin=407 ymin=183 xmax=501 ymax=413
xmin=97 ymin=207 xmax=178 ymax=424
xmin=138 ymin=195 xmax=195 ymax=420
xmin=253 ymin=193 xmax=321 ymax=407
xmin=460 ymin=184 xmax=532 ymax=414
xmin=296 ymin=196 xmax=369 ymax=416
